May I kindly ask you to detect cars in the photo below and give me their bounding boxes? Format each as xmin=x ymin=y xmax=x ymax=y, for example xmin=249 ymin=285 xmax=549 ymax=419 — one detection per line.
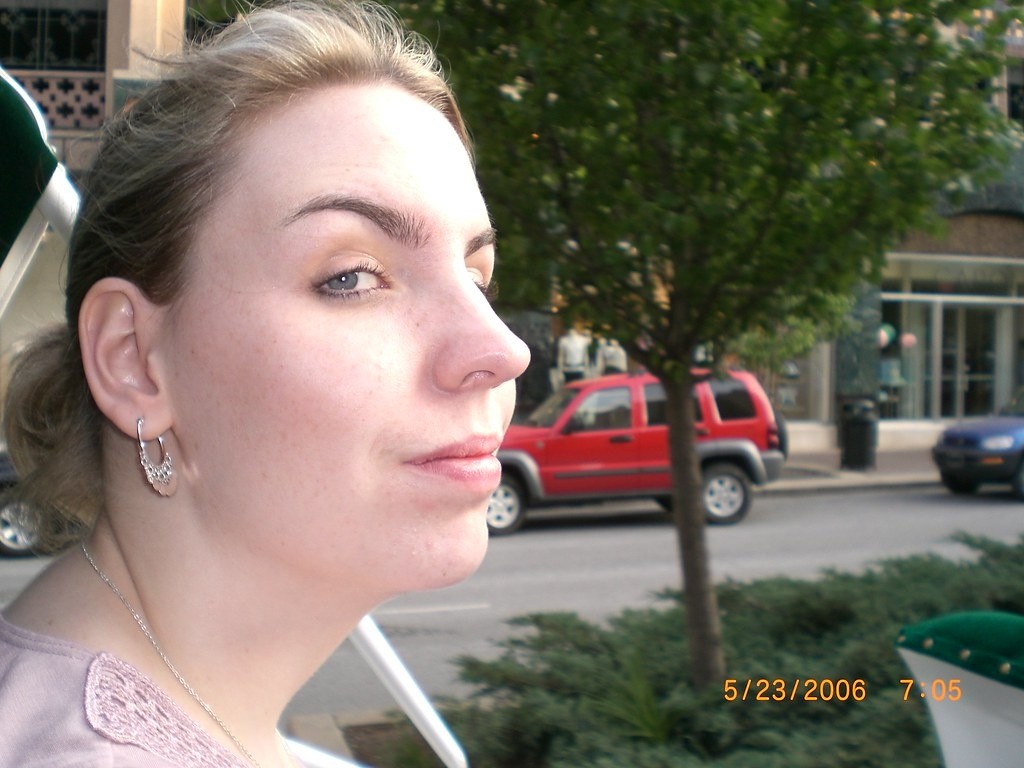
xmin=929 ymin=391 xmax=1024 ymax=504
xmin=878 ymin=359 xmax=906 ymax=414
xmin=0 ymin=442 xmax=93 ymax=563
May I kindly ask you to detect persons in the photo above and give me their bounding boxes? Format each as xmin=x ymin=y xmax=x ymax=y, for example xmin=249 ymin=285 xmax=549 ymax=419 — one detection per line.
xmin=556 ymin=321 xmax=630 ymax=381
xmin=0 ymin=0 xmax=532 ymax=768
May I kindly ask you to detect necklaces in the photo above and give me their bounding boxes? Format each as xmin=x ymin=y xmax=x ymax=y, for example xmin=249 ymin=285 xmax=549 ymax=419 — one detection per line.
xmin=78 ymin=538 xmax=261 ymax=768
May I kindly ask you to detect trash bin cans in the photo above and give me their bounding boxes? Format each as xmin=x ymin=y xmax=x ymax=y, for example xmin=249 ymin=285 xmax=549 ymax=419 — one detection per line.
xmin=840 ymin=398 xmax=879 ymax=470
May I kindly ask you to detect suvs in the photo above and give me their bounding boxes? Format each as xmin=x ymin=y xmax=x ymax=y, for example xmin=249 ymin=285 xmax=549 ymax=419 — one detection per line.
xmin=486 ymin=364 xmax=787 ymax=540
